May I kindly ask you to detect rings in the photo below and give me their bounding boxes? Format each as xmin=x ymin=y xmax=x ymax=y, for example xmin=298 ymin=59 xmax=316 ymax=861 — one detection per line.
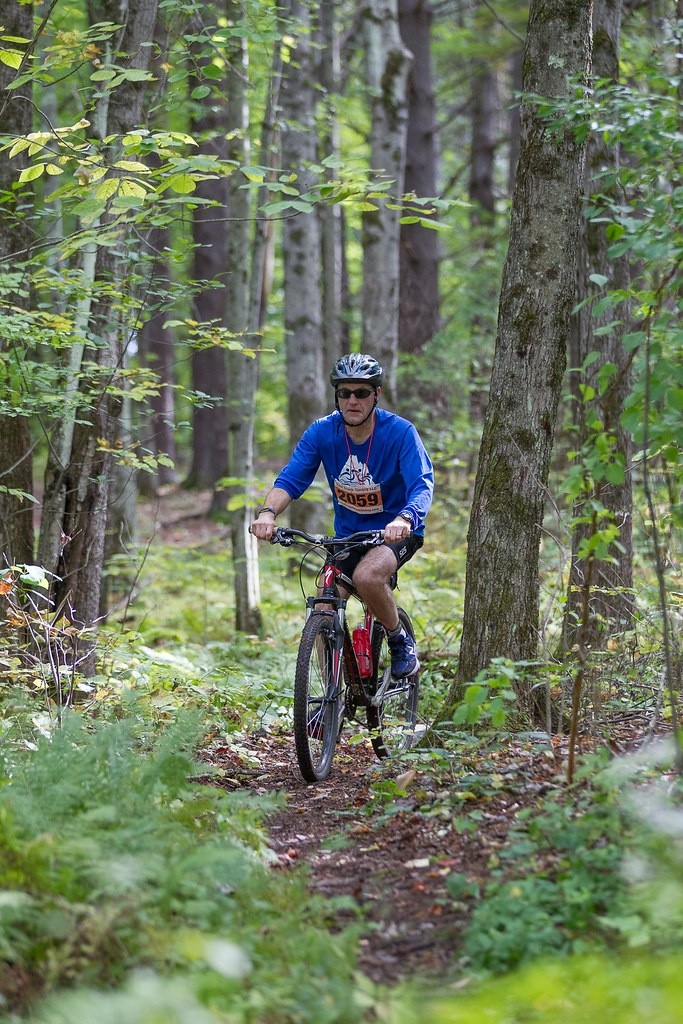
xmin=397 ymin=533 xmax=401 ymax=535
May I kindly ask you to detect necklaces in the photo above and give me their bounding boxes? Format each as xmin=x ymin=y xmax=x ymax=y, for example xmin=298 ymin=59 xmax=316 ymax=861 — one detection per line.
xmin=344 ymin=412 xmax=375 ymax=483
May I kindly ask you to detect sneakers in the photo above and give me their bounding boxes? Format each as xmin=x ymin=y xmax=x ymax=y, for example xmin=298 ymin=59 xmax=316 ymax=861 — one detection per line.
xmin=309 ymin=706 xmax=345 ymax=741
xmin=388 ymin=631 xmax=420 ymax=683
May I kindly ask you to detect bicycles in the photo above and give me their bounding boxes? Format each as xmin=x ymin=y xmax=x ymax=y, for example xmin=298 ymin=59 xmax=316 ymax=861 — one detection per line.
xmin=246 ymin=522 xmax=423 ymax=783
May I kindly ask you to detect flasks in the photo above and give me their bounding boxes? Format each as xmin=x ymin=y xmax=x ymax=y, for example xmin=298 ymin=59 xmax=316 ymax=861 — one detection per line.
xmin=353 ymin=621 xmax=371 ymax=676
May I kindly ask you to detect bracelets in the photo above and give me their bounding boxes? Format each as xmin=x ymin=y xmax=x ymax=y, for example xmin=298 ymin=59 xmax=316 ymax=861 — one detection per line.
xmin=258 ymin=508 xmax=274 ymax=515
xmin=399 ymin=512 xmax=413 ymax=522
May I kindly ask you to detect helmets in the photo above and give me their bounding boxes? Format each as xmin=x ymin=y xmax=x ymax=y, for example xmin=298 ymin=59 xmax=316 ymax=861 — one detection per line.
xmin=330 ymin=353 xmax=383 ymax=386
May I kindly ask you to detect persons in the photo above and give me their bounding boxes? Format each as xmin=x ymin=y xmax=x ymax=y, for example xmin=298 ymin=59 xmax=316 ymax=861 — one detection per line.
xmin=252 ymin=353 xmax=434 ymax=736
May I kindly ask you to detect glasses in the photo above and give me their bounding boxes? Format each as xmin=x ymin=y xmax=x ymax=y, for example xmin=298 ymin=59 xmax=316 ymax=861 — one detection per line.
xmin=337 ymin=387 xmax=375 ymax=400
